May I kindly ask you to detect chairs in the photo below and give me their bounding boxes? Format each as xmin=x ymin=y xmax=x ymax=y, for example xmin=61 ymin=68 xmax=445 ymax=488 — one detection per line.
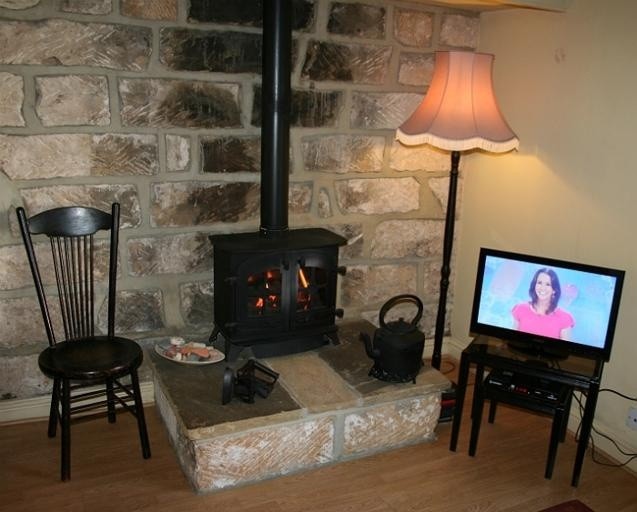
xmin=16 ymin=203 xmax=152 ymax=481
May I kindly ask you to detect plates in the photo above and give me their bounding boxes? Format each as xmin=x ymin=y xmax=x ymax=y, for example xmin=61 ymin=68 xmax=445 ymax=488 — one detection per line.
xmin=154 ymin=339 xmax=227 ymax=369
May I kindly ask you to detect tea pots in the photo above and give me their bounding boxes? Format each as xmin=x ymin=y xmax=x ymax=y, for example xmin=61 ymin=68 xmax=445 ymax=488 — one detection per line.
xmin=358 ymin=293 xmax=427 ymax=385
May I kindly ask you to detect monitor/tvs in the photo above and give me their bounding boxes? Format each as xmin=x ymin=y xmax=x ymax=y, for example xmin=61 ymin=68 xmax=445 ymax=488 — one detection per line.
xmin=470 ymin=247 xmax=626 ymax=363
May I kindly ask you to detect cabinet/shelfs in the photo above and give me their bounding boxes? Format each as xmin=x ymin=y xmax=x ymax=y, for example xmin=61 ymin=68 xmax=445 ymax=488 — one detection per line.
xmin=448 ymin=336 xmax=604 ymax=488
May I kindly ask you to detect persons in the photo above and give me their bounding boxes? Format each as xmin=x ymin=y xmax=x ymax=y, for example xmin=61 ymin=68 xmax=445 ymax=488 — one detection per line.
xmin=510 ymin=266 xmax=574 ymax=341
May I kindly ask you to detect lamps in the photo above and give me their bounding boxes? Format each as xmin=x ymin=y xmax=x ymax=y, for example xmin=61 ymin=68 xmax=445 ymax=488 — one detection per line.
xmin=397 ymin=50 xmax=518 ymax=422
